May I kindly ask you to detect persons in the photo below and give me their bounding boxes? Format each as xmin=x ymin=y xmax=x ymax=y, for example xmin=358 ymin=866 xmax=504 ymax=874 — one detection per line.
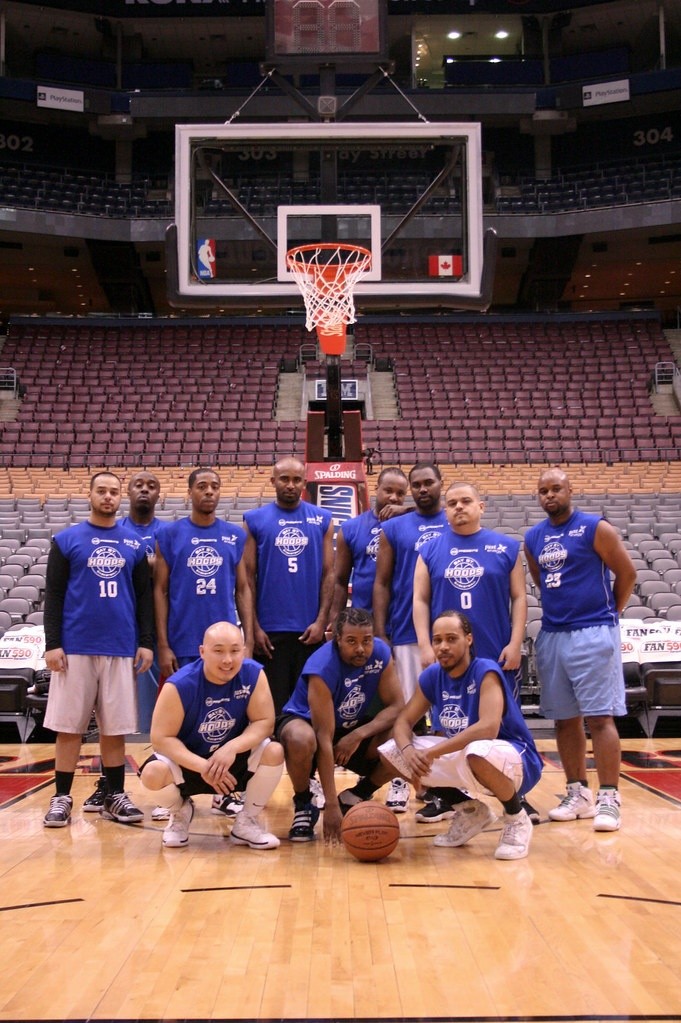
xmin=273 ymin=606 xmax=405 ymax=842
xmin=42 ymin=472 xmax=153 ymax=828
xmin=410 ymin=482 xmax=543 ymax=826
xmin=150 ymin=467 xmax=253 ymax=820
xmin=330 ymin=461 xmax=449 ymax=813
xmin=376 ymin=610 xmax=544 ymax=860
xmin=522 ymin=469 xmax=638 ymax=832
xmin=136 ymin=621 xmax=285 ymax=850
xmin=82 ymin=470 xmax=175 ymax=812
xmin=240 ymin=457 xmax=335 ymax=809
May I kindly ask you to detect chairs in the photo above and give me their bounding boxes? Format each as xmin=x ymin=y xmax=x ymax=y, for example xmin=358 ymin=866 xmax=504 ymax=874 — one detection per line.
xmin=0 ymin=161 xmax=681 ymax=221
xmin=1 ymin=327 xmax=681 ymax=741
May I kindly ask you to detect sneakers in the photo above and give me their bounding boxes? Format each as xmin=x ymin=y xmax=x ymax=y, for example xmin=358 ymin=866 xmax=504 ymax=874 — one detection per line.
xmin=82 ymin=780 xmax=107 ymax=811
xmin=309 ymin=778 xmax=325 ymax=809
xmin=152 ymin=804 xmax=170 ymax=819
xmin=289 ymin=806 xmax=318 ymax=842
xmin=163 ymin=799 xmax=194 ymax=847
xmin=211 ymin=794 xmax=245 ymax=817
xmin=495 ymin=811 xmax=533 ymax=859
xmin=385 ymin=778 xmax=410 ymax=811
xmin=44 ymin=795 xmax=72 ymax=826
xmin=230 ymin=811 xmax=280 ymax=849
xmin=593 ymin=790 xmax=621 ymax=830
xmin=104 ymin=790 xmax=144 ymax=822
xmin=415 ymin=799 xmax=456 ymax=823
xmin=337 ymin=788 xmax=373 ymax=817
xmin=548 ymin=782 xmax=595 ymax=820
xmin=432 ymin=802 xmax=495 ymax=847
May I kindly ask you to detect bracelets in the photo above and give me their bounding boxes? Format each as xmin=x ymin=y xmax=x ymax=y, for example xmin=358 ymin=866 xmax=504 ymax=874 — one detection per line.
xmin=401 ymin=744 xmax=413 ymax=752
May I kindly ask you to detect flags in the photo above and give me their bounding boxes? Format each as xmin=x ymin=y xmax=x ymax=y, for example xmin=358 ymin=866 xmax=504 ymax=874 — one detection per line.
xmin=429 ymin=255 xmax=462 ymax=276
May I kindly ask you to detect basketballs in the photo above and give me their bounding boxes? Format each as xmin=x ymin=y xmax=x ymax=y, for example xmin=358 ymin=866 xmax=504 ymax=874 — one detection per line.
xmin=340 ymin=800 xmax=401 ymax=861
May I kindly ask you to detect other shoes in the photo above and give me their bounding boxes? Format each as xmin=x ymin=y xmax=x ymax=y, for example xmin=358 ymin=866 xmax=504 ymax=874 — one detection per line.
xmin=520 ymin=801 xmax=539 ymax=821
